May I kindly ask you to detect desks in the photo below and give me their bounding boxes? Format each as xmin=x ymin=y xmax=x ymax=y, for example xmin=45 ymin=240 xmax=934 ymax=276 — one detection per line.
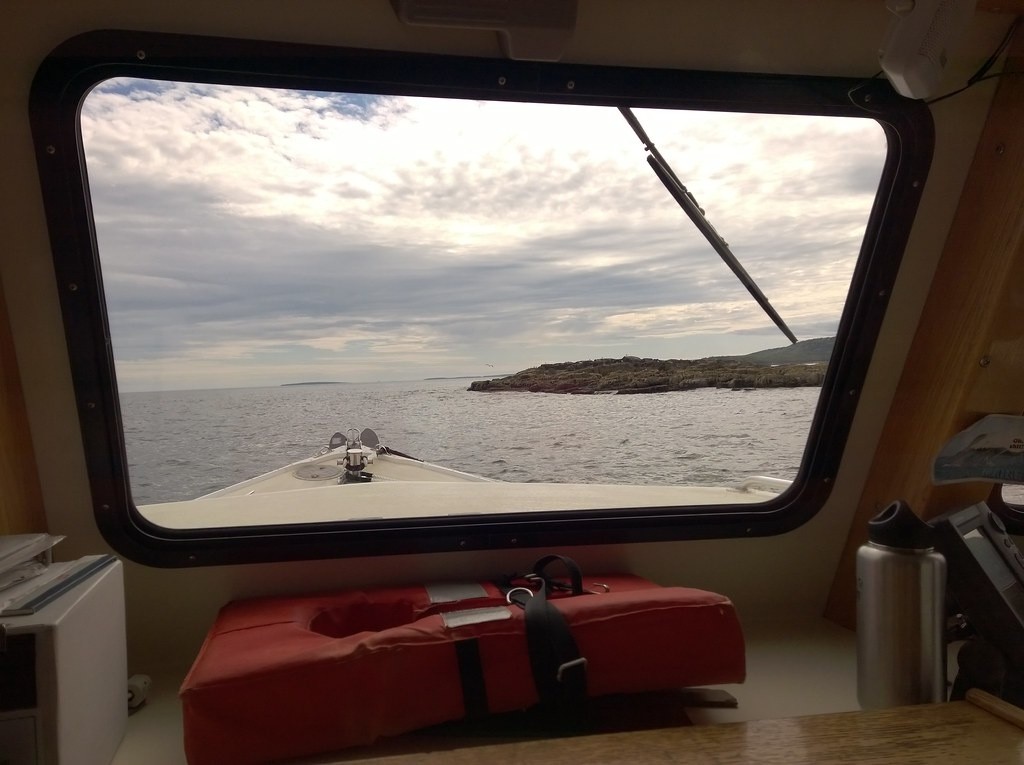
xmin=0 ymin=558 xmax=127 ymax=765
xmin=324 ymin=689 xmax=1024 ymax=765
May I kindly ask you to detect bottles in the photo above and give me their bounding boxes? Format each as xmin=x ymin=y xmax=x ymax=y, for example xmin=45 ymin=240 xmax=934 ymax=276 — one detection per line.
xmin=855 ymin=538 xmax=948 ymax=710
